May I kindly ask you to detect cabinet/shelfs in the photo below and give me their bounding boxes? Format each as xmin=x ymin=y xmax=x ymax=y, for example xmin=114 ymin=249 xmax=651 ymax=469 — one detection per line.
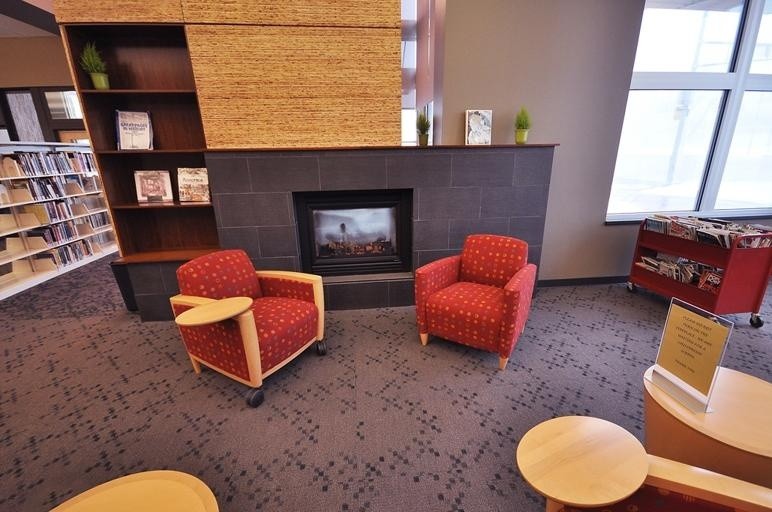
xmin=626 ymin=217 xmax=772 ymax=328
xmin=54 ymin=0 xmax=222 ymax=322
xmin=0 ymin=142 xmax=119 ymax=301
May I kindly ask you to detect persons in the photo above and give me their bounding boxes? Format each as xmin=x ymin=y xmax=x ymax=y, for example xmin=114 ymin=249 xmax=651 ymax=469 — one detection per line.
xmin=468 ymin=111 xmax=490 ymax=145
xmin=140 ymin=171 xmax=167 ymax=202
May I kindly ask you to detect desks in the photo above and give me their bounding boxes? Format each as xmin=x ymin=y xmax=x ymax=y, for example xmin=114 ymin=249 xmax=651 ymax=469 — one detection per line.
xmin=643 ymin=363 xmax=771 ymax=490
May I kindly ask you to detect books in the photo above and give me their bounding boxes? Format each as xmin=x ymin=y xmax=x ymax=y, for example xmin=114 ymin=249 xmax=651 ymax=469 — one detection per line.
xmin=134 ymin=170 xmax=173 ymax=203
xmin=118 ymin=111 xmax=150 ymax=149
xmin=1 ymin=150 xmax=114 ymax=269
xmin=635 ymin=212 xmax=772 ymax=296
xmin=178 ymin=168 xmax=210 ymax=203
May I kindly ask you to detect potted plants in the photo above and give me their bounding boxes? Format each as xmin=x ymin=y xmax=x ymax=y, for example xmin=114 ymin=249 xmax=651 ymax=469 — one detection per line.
xmin=78 ymin=40 xmax=110 ymax=90
xmin=416 ymin=113 xmax=430 ymax=147
xmin=515 ymin=109 xmax=530 ymax=145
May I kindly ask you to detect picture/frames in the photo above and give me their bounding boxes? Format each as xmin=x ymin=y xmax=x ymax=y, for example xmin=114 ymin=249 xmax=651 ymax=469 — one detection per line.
xmin=116 ymin=108 xmax=154 ymax=152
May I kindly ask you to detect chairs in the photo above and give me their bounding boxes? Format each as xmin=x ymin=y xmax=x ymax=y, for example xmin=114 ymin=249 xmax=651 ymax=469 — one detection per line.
xmin=415 ymin=234 xmax=537 ymax=369
xmin=169 ymin=250 xmax=327 ymax=408
